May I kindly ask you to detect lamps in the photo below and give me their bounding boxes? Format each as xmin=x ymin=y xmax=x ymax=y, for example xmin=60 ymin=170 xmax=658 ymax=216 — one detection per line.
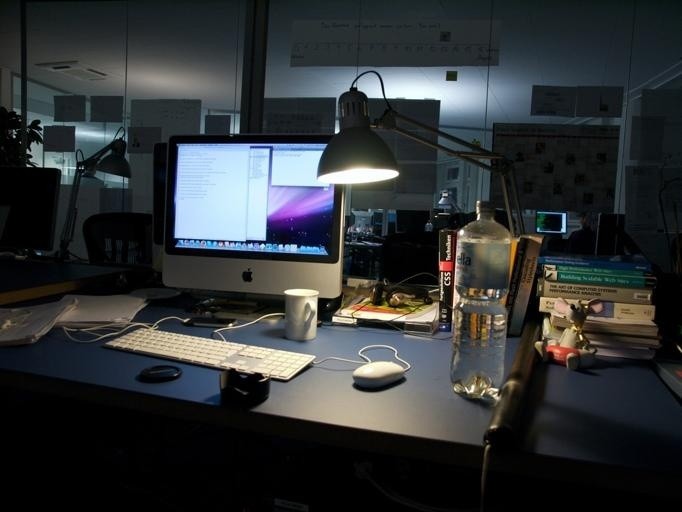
xmin=51 ymin=136 xmax=133 ymax=262
xmin=314 ymin=90 xmax=523 ymax=236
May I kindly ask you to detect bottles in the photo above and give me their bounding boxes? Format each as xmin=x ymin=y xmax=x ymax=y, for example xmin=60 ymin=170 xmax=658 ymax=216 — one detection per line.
xmin=425 ymin=218 xmax=433 ymax=241
xmin=438 ymin=190 xmax=454 ymax=214
xmin=453 ymin=201 xmax=511 ymax=400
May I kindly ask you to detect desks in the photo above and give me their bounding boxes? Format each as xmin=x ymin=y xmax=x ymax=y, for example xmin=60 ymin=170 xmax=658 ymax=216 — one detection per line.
xmin=1 ymin=286 xmax=682 ymax=511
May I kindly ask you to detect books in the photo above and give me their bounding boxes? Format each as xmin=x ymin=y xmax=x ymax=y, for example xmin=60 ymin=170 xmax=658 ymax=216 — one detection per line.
xmin=335 ymin=277 xmax=439 ymax=323
xmin=506 ymin=234 xmax=662 ymax=362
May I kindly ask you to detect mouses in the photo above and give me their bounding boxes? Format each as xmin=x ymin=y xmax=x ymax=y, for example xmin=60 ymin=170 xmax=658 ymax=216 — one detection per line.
xmin=352 ymin=361 xmax=405 ymax=388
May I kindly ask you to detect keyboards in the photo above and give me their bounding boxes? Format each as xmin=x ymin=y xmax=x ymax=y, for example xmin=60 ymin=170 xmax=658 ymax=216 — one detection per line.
xmin=106 ymin=327 xmax=317 ymax=382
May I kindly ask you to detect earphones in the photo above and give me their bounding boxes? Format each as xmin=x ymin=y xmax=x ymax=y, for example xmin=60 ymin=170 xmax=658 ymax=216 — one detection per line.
xmin=2 ymin=302 xmax=35 ymax=331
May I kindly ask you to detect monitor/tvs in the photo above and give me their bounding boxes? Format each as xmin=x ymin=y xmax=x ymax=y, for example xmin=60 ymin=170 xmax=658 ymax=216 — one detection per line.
xmin=535 ymin=212 xmax=568 ymax=235
xmin=153 ymin=143 xmax=167 ymax=271
xmin=0 ymin=167 xmax=61 ymax=252
xmin=162 ymin=134 xmax=344 ymax=327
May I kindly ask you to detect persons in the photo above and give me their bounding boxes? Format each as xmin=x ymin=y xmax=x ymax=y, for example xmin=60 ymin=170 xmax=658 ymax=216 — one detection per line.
xmin=79 ymin=160 xmax=106 ymax=186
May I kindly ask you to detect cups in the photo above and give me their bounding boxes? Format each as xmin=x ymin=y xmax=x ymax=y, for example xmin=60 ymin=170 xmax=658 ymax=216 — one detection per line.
xmin=284 ymin=289 xmax=320 ymax=342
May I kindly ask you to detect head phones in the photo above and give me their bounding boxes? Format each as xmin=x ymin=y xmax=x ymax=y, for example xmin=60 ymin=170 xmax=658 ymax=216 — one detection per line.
xmin=370 ymin=281 xmax=427 ymax=307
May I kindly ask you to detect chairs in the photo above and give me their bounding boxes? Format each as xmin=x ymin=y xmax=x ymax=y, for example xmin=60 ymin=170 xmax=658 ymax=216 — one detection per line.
xmin=82 ymin=210 xmax=154 ymax=267
